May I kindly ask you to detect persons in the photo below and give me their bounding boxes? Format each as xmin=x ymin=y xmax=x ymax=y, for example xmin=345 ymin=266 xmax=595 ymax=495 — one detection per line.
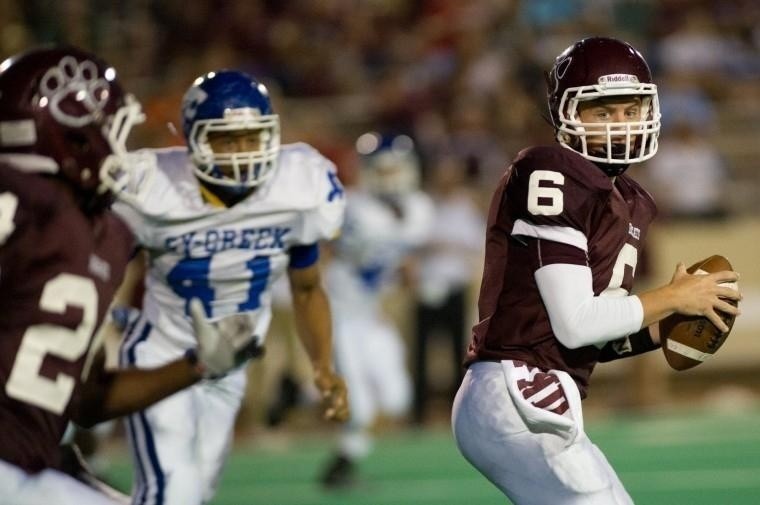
xmin=105 ymin=64 xmax=353 ymax=505
xmin=1 ymin=45 xmax=258 ymax=505
xmin=1 ymin=1 xmax=756 ymax=489
xmin=448 ymin=32 xmax=743 ymax=502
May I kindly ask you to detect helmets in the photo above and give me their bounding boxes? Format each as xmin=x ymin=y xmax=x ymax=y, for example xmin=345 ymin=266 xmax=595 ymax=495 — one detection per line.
xmin=0 ymin=43 xmax=131 ymax=208
xmin=182 ymin=69 xmax=275 ymax=152
xmin=545 ymin=34 xmax=658 ymax=136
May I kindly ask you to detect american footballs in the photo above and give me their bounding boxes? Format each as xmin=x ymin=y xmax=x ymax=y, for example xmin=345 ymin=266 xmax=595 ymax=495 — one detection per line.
xmin=659 ymin=256 xmax=737 ymax=370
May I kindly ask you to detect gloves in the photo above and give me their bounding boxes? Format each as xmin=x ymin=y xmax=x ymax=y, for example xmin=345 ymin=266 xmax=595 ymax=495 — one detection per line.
xmin=188 ymin=298 xmax=263 ymax=382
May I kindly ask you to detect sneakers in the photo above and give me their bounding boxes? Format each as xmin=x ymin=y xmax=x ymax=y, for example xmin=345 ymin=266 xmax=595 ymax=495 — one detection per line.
xmin=321 ymin=456 xmax=356 ymax=487
xmin=266 ymin=362 xmax=303 ymax=429
